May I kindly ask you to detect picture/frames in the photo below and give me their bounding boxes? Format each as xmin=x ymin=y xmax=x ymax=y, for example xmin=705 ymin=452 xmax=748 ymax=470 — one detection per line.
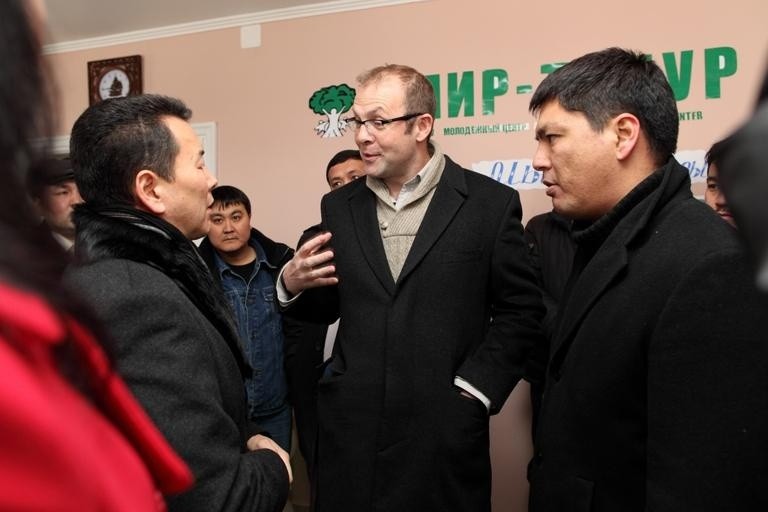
xmin=27 ymin=119 xmax=216 ymax=246
xmin=86 ymin=55 xmax=144 ymax=108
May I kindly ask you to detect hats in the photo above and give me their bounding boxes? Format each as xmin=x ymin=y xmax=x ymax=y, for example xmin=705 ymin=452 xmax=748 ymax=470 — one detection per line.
xmin=27 ymin=155 xmax=75 ymax=196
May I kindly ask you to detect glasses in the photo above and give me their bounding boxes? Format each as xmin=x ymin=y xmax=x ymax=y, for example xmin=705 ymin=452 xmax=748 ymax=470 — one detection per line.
xmin=345 ymin=113 xmax=423 ymax=131
